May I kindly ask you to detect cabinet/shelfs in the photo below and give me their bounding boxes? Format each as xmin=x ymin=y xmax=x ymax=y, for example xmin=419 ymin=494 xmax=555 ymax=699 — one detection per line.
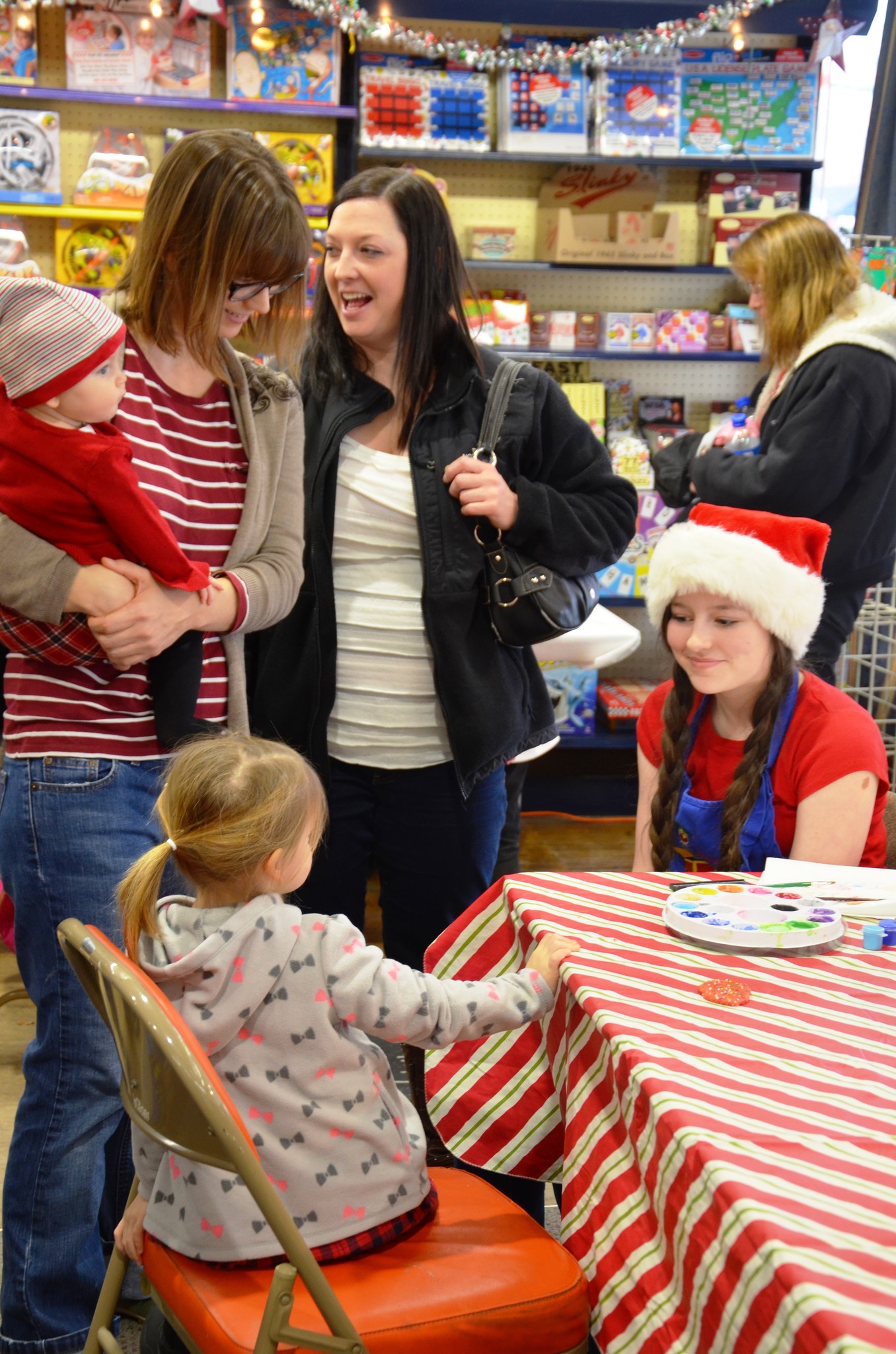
xmin=347 ymin=115 xmax=826 ymax=813
xmin=0 ymin=0 xmax=365 ymax=245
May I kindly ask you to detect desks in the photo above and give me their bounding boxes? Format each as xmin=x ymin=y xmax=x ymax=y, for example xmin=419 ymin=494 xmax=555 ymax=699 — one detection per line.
xmin=412 ymin=864 xmax=896 ymax=1354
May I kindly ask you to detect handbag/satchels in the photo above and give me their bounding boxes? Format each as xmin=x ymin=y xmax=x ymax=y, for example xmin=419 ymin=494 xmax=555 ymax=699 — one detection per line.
xmin=470 ymin=357 xmax=601 ymax=650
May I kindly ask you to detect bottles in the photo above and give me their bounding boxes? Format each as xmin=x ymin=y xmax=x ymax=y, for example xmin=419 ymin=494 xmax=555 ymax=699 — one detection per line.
xmin=723 ymin=410 xmax=766 ymax=464
xmin=735 ymin=396 xmax=757 ymax=422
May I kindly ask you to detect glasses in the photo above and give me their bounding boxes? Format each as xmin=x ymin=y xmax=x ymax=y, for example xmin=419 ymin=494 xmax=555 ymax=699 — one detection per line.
xmin=228 ymin=271 xmax=304 ymax=302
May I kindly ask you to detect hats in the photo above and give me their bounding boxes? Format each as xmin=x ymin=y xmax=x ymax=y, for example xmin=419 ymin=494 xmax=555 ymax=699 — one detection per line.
xmin=643 ymin=502 xmax=832 ymax=662
xmin=0 ymin=276 xmax=128 ymax=409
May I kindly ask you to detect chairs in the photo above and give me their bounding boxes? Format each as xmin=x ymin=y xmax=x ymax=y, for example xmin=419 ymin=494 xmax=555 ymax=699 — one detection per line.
xmin=55 ymin=917 xmax=593 ymax=1353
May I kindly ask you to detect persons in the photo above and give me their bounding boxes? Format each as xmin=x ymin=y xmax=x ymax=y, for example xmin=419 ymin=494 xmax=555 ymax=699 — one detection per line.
xmin=0 ymin=272 xmax=232 ymax=750
xmin=631 ymin=501 xmax=893 ymax=876
xmin=0 ymin=127 xmax=311 ymax=1354
xmin=263 ymin=164 xmax=634 ymax=1166
xmin=649 ymin=210 xmax=896 ymax=686
xmin=112 ymin=732 xmax=585 ymax=1273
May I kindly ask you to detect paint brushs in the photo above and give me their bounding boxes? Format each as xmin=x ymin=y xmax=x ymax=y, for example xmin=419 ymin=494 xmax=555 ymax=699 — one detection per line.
xmin=757 ymin=881 xmax=836 ymax=888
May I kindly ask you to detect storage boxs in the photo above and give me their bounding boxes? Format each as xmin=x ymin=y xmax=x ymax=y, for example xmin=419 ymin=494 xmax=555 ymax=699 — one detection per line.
xmin=53 ymin=216 xmax=140 ymax=289
xmin=1 ymin=0 xmax=38 ymax=88
xmin=63 ymin=2 xmax=213 ymax=101
xmin=253 ymin=129 xmax=332 ymax=231
xmin=336 ymin=45 xmax=830 ymax=733
xmin=0 ymin=108 xmax=63 ymax=207
xmin=221 ymin=0 xmax=342 ymax=110
xmin=72 ymin=127 xmax=157 ymax=208
